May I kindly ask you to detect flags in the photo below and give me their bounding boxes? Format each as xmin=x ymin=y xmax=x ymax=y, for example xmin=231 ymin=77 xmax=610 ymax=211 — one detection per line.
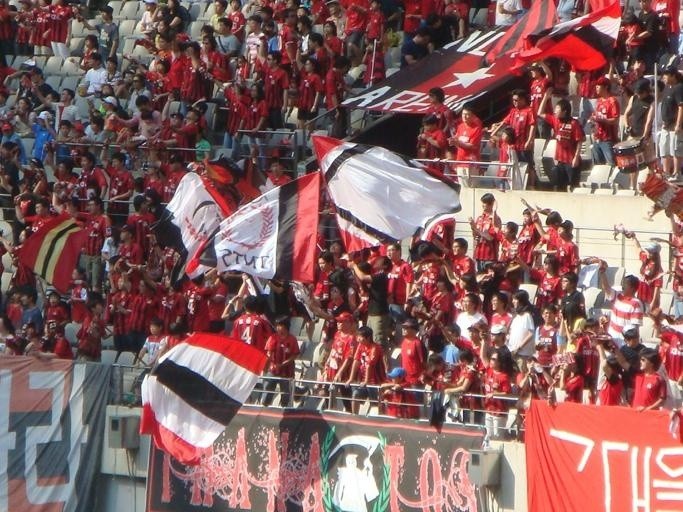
xmin=513 ymin=1 xmax=623 ymax=72
xmin=183 ymin=169 xmax=320 ymax=283
xmin=148 ymin=159 xmax=261 ymax=263
xmin=312 ymin=136 xmax=462 ymax=258
xmin=137 ymin=331 xmax=270 ymax=467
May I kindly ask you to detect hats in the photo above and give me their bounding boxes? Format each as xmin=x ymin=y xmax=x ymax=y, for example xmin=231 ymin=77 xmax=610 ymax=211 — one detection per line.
xmin=555 ymin=220 xmax=573 ymax=231
xmin=37 ymin=112 xmax=52 ymax=121
xmin=400 ymin=318 xmax=418 ymax=329
xmin=2 ymin=125 xmax=12 ymax=131
xmin=645 ymin=243 xmax=662 ymax=254
xmin=591 ymin=77 xmax=611 ymax=86
xmin=335 ymin=312 xmax=354 ymax=322
xmin=101 ymin=96 xmax=118 ymax=107
xmin=632 ymin=79 xmax=650 ymax=92
xmin=660 ymin=66 xmax=677 ymax=73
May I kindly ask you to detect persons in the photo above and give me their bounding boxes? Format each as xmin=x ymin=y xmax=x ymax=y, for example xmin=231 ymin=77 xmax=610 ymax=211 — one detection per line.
xmin=233 ymin=193 xmax=682 ymax=441
xmin=2 ymin=2 xmax=385 ymax=363
xmin=400 ymin=3 xmax=681 ymax=190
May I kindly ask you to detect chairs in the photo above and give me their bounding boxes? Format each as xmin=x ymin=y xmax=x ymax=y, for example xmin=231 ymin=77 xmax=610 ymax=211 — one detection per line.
xmin=1 ymin=272 xmax=15 ymax=293
xmin=443 ymin=8 xmax=650 ymax=196
xmin=65 ymin=322 xmax=148 ymax=367
xmin=269 ymin=315 xmax=380 ymax=416
xmin=0 ymin=0 xmax=365 ymax=186
xmin=519 ymin=264 xmax=675 ymax=350
xmin=2 ymin=251 xmax=17 ymax=271
